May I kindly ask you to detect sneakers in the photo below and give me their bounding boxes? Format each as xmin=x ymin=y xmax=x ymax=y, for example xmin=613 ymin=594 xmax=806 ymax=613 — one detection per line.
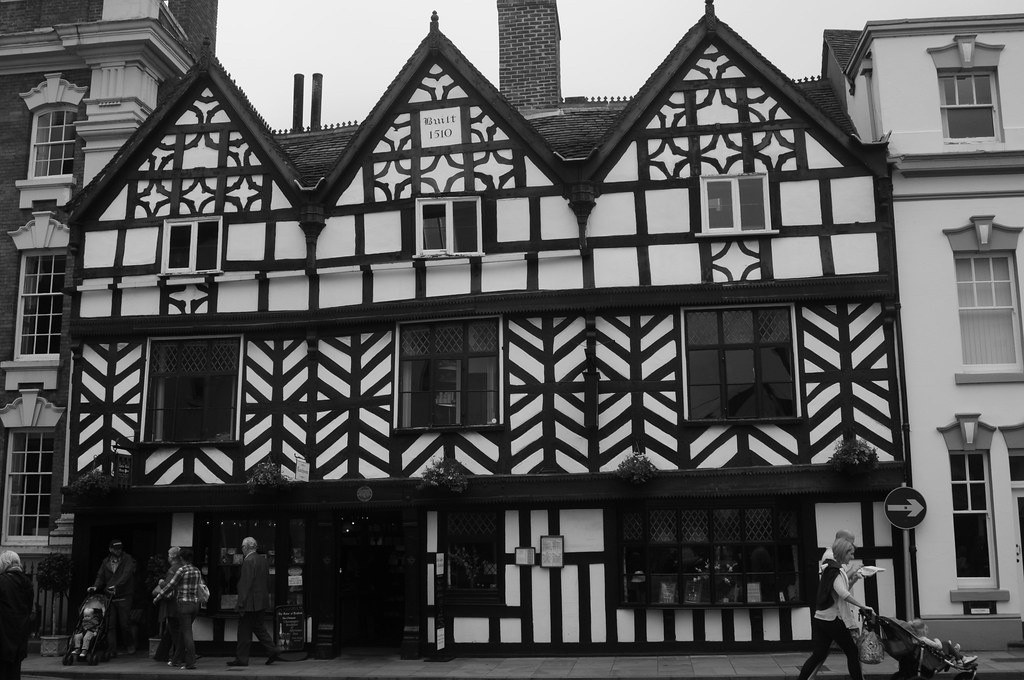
xmin=954 ymin=643 xmax=960 ymax=654
xmin=962 ymin=655 xmax=978 ymax=666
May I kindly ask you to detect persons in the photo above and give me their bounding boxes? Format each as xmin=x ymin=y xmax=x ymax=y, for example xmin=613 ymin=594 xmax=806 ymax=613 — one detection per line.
xmin=87 ymin=540 xmax=204 ymax=670
xmin=681 ymin=547 xmax=775 ymax=599
xmin=72 ymin=608 xmax=98 ymax=656
xmin=0 ymin=549 xmax=35 ymax=680
xmin=798 ymin=530 xmax=978 ymax=680
xmin=226 ymin=537 xmax=280 ymax=666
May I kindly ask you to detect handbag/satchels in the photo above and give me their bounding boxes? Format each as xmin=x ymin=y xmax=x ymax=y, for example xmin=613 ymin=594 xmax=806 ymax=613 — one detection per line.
xmin=197 ymin=583 xmax=211 ymax=605
xmin=855 ymin=627 xmax=885 ymax=664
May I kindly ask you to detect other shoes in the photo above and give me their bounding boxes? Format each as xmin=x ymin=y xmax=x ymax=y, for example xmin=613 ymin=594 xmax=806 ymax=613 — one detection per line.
xmin=71 ymin=648 xmax=81 ymax=654
xmin=127 ymin=644 xmax=137 ymax=655
xmin=168 ymin=660 xmax=186 ymax=667
xmin=265 ymin=652 xmax=281 ymax=665
xmin=226 ymin=658 xmax=249 ymax=666
xmin=106 ymin=650 xmax=117 ymax=658
xmin=79 ymin=650 xmax=87 ymax=657
xmin=180 ymin=664 xmax=196 ymax=670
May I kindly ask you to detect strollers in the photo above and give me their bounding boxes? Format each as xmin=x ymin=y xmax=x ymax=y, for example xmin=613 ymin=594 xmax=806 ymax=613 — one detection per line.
xmin=865 ymin=610 xmax=979 ymax=680
xmin=62 ymin=586 xmax=114 ymax=667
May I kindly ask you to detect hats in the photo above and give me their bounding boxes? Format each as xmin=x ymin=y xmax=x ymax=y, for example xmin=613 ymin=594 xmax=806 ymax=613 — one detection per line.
xmin=109 ymin=540 xmax=124 ymax=549
